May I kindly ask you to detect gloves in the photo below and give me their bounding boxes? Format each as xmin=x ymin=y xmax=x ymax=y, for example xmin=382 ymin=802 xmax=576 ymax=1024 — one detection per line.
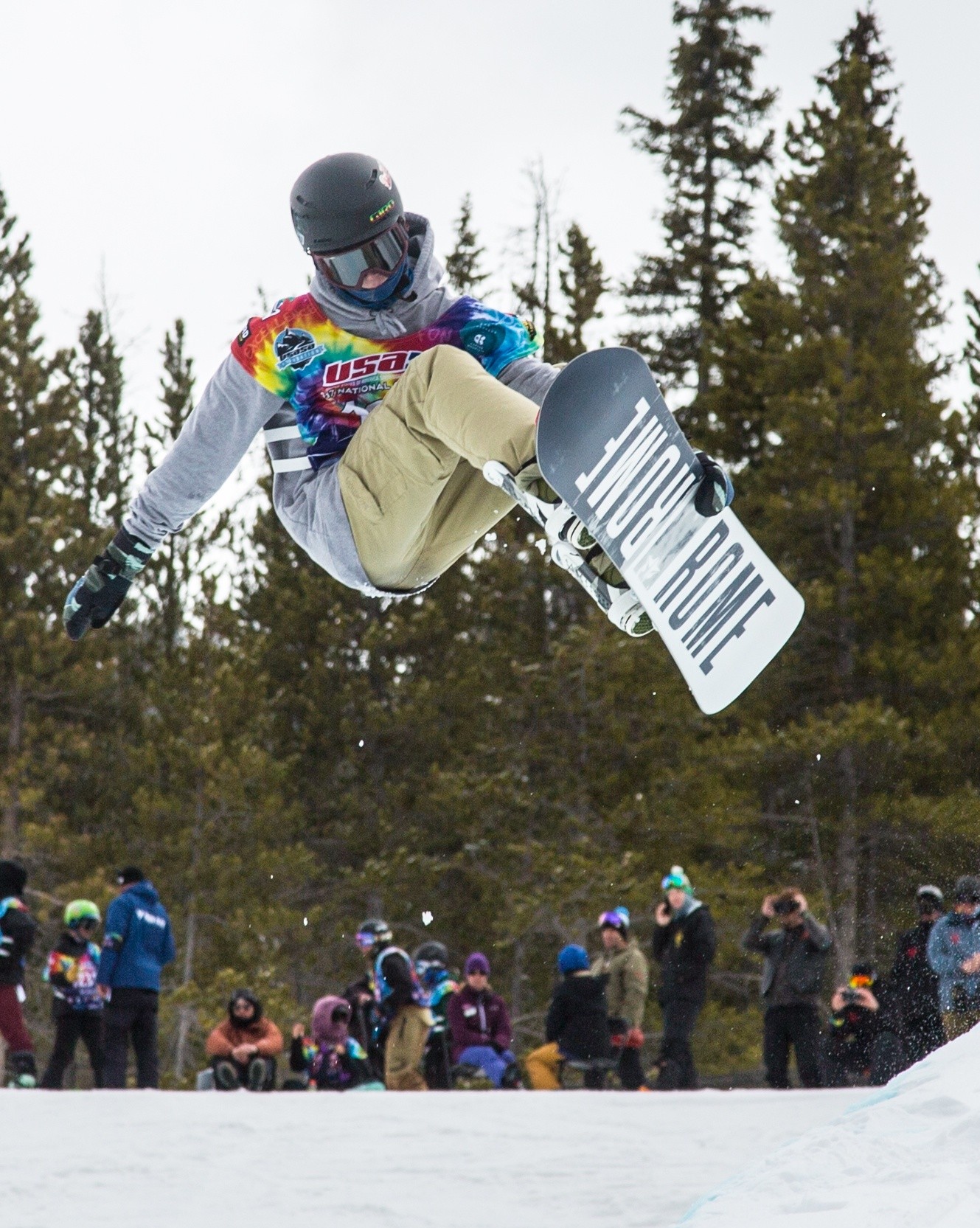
xmin=687 ymin=445 xmax=735 ymax=519
xmin=62 ymin=525 xmax=153 ymax=640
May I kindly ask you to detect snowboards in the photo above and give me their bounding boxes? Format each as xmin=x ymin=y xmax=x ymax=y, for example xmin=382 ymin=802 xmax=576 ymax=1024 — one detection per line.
xmin=535 ymin=347 xmax=805 ymax=716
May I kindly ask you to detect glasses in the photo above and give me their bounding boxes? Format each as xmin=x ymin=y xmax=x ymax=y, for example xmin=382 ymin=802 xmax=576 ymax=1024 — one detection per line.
xmin=597 ymin=912 xmax=622 ymax=928
xmin=661 ymin=874 xmax=684 ymax=890
xmin=311 ymin=220 xmax=409 ymax=290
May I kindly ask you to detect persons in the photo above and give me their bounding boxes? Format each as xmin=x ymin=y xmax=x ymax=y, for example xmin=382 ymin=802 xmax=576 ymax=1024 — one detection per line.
xmin=0 ymin=861 xmax=980 ymax=1091
xmin=64 ymin=153 xmax=734 ymax=639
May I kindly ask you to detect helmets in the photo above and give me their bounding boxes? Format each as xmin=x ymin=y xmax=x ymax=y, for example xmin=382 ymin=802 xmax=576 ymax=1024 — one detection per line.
xmin=354 ymin=919 xmax=392 ymax=946
xmin=414 ymin=941 xmax=448 ymax=976
xmin=289 ymin=153 xmax=403 ymax=255
xmin=917 ymin=884 xmax=943 ymax=901
xmin=955 ymin=876 xmax=980 ymax=898
xmin=557 ymin=944 xmax=590 ymax=972
xmin=63 ymin=899 xmax=102 ymax=925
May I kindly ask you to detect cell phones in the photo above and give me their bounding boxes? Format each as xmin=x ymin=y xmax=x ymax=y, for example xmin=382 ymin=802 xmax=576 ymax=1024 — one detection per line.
xmin=842 ymin=992 xmax=859 ymax=1001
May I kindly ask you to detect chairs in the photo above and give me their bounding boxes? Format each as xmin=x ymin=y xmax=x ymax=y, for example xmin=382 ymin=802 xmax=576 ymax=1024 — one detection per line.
xmin=440 ymin=1026 xmax=476 ymax=1090
xmin=563 ymin=1014 xmax=628 ymax=1091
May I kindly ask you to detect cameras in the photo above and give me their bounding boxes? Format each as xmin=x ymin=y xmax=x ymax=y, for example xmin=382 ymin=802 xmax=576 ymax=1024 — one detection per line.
xmin=774 ymin=899 xmax=798 ymax=914
xmin=920 ymin=901 xmax=935 ymax=914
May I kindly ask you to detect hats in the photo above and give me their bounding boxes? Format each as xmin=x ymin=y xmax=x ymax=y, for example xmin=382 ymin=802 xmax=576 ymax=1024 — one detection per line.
xmin=117 ymin=866 xmax=144 ymax=887
xmin=615 ymin=906 xmax=630 ymax=928
xmin=671 ymin=864 xmax=694 ymax=895
xmin=464 ymin=952 xmax=488 ymax=972
